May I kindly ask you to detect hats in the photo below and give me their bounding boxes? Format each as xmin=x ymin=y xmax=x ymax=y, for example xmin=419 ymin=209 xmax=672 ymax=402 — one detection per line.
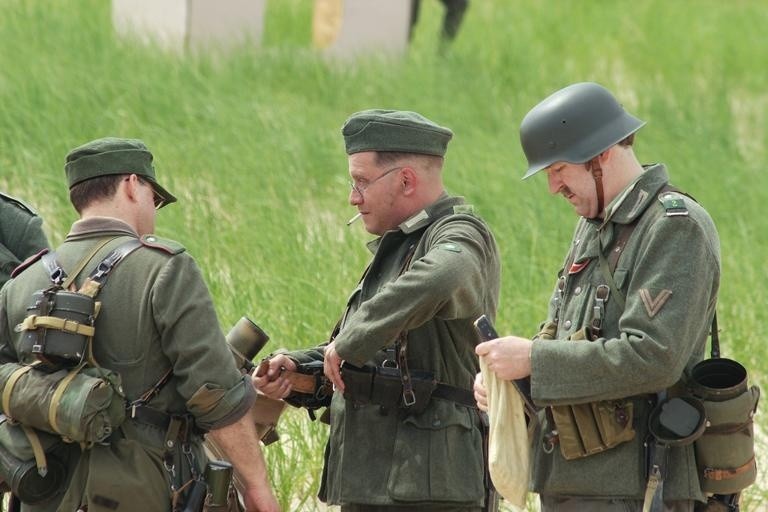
xmin=341 ymin=108 xmax=452 ymax=157
xmin=66 ymin=137 xmax=178 ymax=212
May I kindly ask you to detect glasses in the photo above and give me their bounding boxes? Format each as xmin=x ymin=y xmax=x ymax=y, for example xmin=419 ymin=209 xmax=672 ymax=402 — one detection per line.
xmin=124 ymin=174 xmax=166 ymax=211
xmin=350 ymin=166 xmax=405 ymax=193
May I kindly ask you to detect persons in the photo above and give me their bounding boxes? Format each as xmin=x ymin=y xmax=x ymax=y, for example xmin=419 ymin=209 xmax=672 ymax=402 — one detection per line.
xmin=471 ymin=82 xmax=758 ymax=512
xmin=410 ymin=0 xmax=469 ymax=61
xmin=249 ymin=107 xmax=503 ymax=512
xmin=0 ymin=193 xmax=47 ymax=509
xmin=0 ymin=135 xmax=285 ymax=510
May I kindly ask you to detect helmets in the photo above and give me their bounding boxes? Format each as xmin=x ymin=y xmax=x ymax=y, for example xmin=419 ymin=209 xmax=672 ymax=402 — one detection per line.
xmin=518 ymin=81 xmax=647 ymax=179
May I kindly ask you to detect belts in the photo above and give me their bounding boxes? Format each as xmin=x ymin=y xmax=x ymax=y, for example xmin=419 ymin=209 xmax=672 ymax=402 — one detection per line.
xmin=129 ymin=404 xmax=172 ymax=429
xmin=254 ymin=362 xmax=478 ymax=410
